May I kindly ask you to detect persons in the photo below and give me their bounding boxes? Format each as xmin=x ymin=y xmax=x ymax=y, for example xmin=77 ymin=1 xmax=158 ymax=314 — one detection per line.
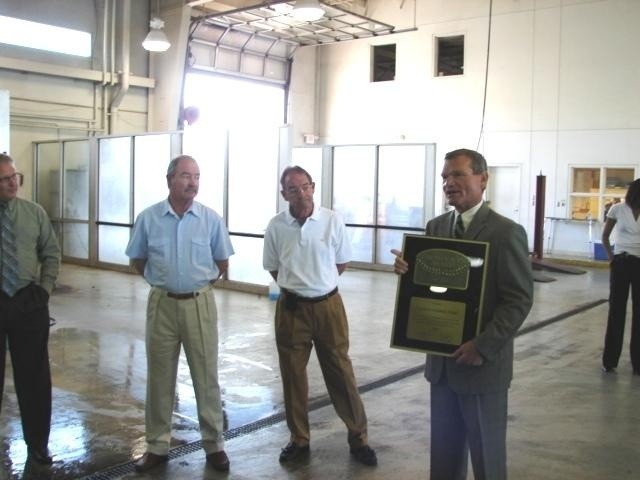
xmin=263 ymin=165 xmax=378 ymax=466
xmin=125 ymin=156 xmax=235 ymax=472
xmin=600 ymin=178 xmax=640 ymax=376
xmin=0 ymin=153 xmax=61 ymax=464
xmin=391 ymin=148 xmax=533 ymax=480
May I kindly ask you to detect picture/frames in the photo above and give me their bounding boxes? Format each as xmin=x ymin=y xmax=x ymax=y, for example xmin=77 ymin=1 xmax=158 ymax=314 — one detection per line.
xmin=389 ymin=232 xmax=490 ymax=358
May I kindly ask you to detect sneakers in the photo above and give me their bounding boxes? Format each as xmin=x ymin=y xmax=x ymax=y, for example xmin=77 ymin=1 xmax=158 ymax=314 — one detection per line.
xmin=350 ymin=445 xmax=377 ymax=466
xmin=280 ymin=441 xmax=309 ymax=462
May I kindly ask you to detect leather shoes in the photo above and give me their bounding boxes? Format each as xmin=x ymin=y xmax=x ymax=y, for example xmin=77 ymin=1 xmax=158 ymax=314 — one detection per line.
xmin=206 ymin=451 xmax=229 ymax=471
xmin=136 ymin=451 xmax=168 ymax=471
xmin=25 ymin=463 xmax=51 ymax=479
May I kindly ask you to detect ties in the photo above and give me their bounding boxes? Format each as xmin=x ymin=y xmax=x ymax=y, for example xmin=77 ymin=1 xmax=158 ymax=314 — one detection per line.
xmin=455 ymin=215 xmax=464 ymax=239
xmin=2 ymin=201 xmax=19 ymax=298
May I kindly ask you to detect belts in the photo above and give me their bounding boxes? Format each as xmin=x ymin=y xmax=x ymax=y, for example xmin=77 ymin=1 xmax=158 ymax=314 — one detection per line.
xmin=164 ymin=292 xmax=201 ymax=299
xmin=279 ymin=287 xmax=338 ymax=302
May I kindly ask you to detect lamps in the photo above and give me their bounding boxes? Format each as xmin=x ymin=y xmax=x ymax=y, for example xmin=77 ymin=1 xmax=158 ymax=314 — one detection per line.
xmin=288 ymin=0 xmax=327 ymax=22
xmin=141 ymin=0 xmax=171 ymax=52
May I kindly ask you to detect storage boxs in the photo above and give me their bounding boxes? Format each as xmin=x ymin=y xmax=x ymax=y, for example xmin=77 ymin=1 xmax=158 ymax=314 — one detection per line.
xmin=594 ymin=239 xmax=615 ymax=261
